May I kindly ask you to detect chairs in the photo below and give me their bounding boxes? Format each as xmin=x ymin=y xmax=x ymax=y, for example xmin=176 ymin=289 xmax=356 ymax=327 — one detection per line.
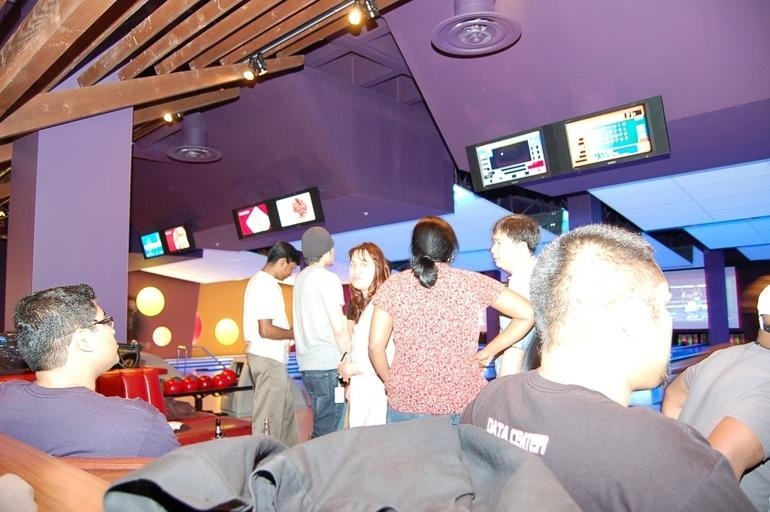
xmin=0 ymin=434 xmax=158 ymax=512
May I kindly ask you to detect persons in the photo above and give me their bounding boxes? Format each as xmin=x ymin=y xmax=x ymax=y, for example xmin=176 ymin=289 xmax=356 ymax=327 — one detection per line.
xmin=242 ymin=240 xmax=300 ymax=447
xmin=662 ymin=283 xmax=770 ymax=512
xmin=0 ymin=283 xmax=182 ymax=458
xmin=462 ymin=222 xmax=758 ymax=512
xmin=291 ymin=226 xmax=349 ymax=438
xmin=337 ymin=241 xmax=395 ymax=428
xmin=491 ymin=214 xmax=541 ymax=378
xmin=128 ymin=295 xmax=142 ymax=351
xmin=368 ymin=215 xmax=535 ymax=426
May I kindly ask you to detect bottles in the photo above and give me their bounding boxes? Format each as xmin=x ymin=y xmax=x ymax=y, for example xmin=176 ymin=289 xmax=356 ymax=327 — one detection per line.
xmin=215 ymin=418 xmax=223 ymax=440
xmin=262 ymin=418 xmax=270 ymax=437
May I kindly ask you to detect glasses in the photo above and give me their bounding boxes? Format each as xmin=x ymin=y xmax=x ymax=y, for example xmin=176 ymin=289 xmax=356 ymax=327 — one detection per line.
xmin=91 ymin=315 xmax=113 ymax=329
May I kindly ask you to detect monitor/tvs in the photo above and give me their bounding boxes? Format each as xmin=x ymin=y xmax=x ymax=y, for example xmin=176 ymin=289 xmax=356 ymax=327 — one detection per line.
xmin=554 ymin=94 xmax=671 ymax=176
xmin=464 ymin=124 xmax=553 ymax=194
xmin=231 ymin=199 xmax=276 ymax=240
xmin=138 ymin=230 xmax=166 ymax=260
xmin=162 ymin=222 xmax=197 ymax=254
xmin=270 ymin=185 xmax=326 ymax=231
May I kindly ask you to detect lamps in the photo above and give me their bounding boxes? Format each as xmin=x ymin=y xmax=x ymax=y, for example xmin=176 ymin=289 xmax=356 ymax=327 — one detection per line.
xmin=348 ymin=0 xmax=379 ymax=25
xmin=243 ymin=51 xmax=268 ymax=80
xmin=214 ymin=317 xmax=240 ymax=346
xmin=164 ymin=112 xmax=183 ymax=122
xmin=135 ymin=286 xmax=166 ymax=317
xmin=151 ymin=325 xmax=173 ymax=348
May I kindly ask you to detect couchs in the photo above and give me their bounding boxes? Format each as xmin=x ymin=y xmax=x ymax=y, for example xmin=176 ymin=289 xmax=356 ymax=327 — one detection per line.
xmin=0 ymin=367 xmax=251 ymax=446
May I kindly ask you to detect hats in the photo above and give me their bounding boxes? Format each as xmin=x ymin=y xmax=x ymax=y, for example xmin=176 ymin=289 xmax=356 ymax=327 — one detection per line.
xmin=301 ymin=227 xmax=334 ymax=258
